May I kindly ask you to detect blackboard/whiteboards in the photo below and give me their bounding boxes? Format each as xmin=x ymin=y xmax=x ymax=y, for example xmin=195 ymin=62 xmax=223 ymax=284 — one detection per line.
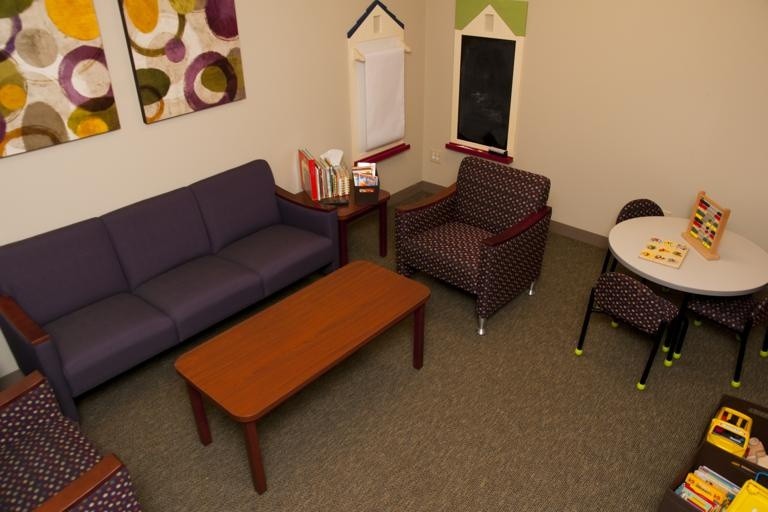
xmin=448 ymin=29 xmax=525 ymax=158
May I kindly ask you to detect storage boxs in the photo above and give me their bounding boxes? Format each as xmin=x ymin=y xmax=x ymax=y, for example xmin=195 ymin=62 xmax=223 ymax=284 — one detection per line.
xmin=657 ymin=393 xmax=768 ymax=512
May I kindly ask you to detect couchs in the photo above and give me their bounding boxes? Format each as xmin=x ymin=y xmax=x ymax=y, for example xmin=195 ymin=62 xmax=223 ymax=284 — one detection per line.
xmin=0 ymin=159 xmax=342 ymax=418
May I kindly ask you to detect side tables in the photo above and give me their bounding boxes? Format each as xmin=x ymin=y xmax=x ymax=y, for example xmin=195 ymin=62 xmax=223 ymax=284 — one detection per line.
xmin=294 ymin=178 xmax=391 ymax=267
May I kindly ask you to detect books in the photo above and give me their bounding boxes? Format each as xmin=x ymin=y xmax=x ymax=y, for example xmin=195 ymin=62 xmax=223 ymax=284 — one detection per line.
xmin=298 ymin=147 xmax=377 ymax=200
xmin=674 ymin=463 xmax=743 ymax=512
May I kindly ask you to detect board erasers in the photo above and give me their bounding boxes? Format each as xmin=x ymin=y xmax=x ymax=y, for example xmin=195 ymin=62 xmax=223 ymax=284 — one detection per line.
xmin=489 ymin=146 xmax=507 ymax=156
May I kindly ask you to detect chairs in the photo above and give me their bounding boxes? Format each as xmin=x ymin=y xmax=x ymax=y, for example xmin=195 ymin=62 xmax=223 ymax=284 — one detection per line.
xmin=393 ymin=157 xmax=553 ymax=337
xmin=574 ymin=198 xmax=768 ymax=391
xmin=0 ymin=369 xmax=144 ymax=512
